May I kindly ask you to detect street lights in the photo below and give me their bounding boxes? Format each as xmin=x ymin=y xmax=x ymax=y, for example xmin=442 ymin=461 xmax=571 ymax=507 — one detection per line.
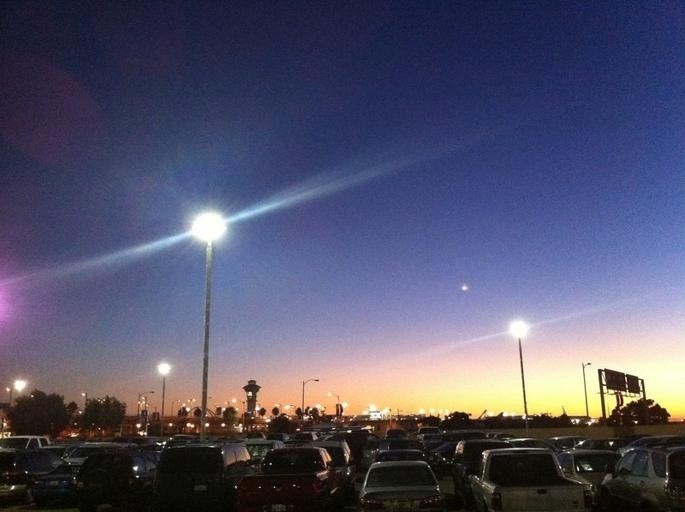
xmin=81 ymin=392 xmax=87 ymax=408
xmin=191 ymin=209 xmax=226 ymax=447
xmin=159 ymin=362 xmax=172 ymax=439
xmin=508 ymin=319 xmax=533 ymax=435
xmin=583 ymin=361 xmax=591 ymax=419
xmin=173 ymin=392 xmax=449 ymax=424
xmin=301 ymin=378 xmax=320 ymax=414
xmin=137 ymin=391 xmax=155 ymax=415
xmin=6 ymin=388 xmax=12 ymax=405
